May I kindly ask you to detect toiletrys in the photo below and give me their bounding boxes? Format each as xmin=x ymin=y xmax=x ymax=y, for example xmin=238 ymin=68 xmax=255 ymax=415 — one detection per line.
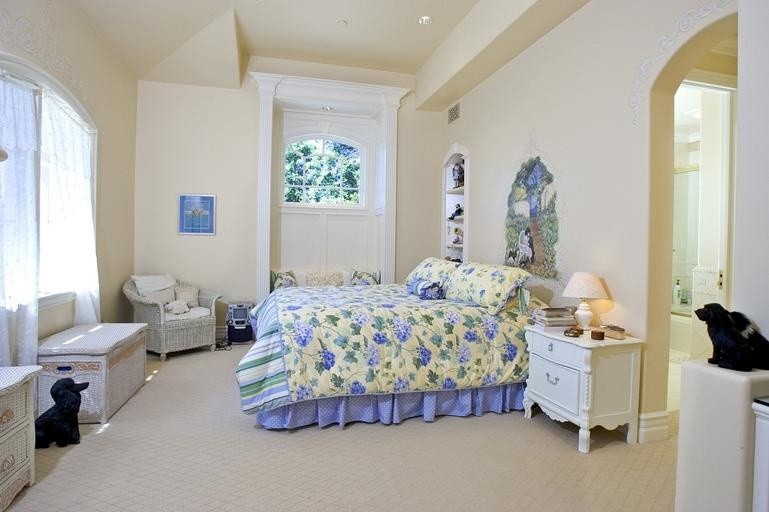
xmin=672 ymin=279 xmax=682 ymax=305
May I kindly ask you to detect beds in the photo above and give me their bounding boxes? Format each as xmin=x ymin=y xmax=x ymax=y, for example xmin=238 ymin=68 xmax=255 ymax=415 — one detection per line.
xmin=234 ymin=256 xmax=550 ymax=432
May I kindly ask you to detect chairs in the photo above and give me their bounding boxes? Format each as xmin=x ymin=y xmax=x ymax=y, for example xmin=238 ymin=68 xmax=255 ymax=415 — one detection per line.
xmin=122 ymin=271 xmax=224 ymax=362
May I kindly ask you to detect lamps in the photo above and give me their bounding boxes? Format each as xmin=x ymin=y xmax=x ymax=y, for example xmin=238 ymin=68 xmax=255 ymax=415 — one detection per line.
xmin=560 ymin=271 xmax=610 ymax=330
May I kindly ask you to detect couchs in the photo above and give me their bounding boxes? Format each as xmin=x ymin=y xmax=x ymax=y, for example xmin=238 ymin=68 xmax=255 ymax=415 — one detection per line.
xmin=271 ymin=267 xmax=381 ymax=290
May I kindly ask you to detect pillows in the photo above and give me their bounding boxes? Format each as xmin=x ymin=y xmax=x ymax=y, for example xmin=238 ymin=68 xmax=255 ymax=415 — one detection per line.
xmin=405 ymin=256 xmax=461 ymax=290
xmin=407 ymin=274 xmax=443 ymax=299
xmin=437 ymin=262 xmax=530 ymax=316
xmin=174 ymin=284 xmax=201 ymax=307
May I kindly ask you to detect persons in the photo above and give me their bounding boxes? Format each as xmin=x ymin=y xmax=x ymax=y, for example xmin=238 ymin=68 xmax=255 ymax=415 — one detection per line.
xmin=522 ymin=227 xmax=532 ymax=264
xmin=448 ymin=204 xmax=461 ymax=220
xmin=452 ymin=227 xmax=462 ymax=243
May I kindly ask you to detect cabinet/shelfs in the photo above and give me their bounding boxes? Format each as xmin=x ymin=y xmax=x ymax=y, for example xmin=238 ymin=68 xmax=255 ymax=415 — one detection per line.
xmin=23 ymin=318 xmax=150 ymax=425
xmin=0 ymin=363 xmax=46 ymax=511
xmin=445 ymin=153 xmax=464 ymax=260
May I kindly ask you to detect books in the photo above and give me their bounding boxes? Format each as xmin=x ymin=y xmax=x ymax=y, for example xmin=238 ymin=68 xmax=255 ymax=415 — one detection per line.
xmin=534 ymin=307 xmax=578 ymax=335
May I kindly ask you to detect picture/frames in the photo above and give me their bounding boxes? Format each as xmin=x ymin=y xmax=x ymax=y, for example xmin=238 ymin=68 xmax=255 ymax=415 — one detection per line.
xmin=178 ymin=193 xmax=216 ymax=237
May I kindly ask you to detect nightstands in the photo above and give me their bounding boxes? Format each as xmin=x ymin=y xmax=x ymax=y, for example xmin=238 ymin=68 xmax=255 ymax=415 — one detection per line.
xmin=523 ymin=320 xmax=647 ymax=454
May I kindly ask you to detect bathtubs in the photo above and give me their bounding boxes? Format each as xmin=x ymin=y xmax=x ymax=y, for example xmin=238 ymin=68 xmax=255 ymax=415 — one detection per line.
xmin=671 ymin=305 xmax=692 ymax=354
